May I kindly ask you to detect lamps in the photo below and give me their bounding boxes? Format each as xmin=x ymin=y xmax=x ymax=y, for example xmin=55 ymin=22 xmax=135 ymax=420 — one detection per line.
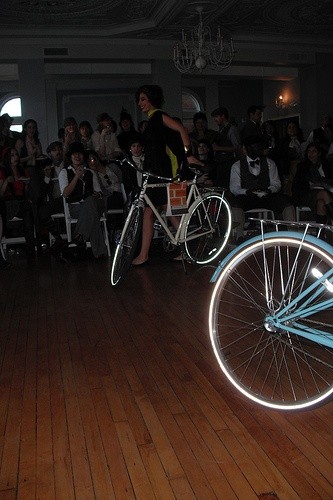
xmin=275 ymin=94 xmax=297 ymax=109
xmin=170 ymin=6 xmax=235 ymax=74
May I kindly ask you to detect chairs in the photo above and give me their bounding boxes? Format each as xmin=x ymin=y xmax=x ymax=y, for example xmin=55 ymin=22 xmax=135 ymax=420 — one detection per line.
xmin=0 ymin=178 xmax=197 ymax=261
xmin=246 ymin=206 xmax=311 ymax=227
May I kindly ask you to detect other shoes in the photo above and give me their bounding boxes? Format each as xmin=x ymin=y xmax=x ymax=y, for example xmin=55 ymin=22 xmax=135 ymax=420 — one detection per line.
xmin=129 ymin=259 xmax=149 ymax=267
xmin=62 ymin=241 xmax=81 ymax=266
xmin=169 ymin=256 xmax=187 ymax=263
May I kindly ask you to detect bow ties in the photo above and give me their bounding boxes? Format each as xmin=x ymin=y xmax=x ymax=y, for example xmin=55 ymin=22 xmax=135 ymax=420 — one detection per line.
xmin=250 ymin=158 xmax=260 ymax=168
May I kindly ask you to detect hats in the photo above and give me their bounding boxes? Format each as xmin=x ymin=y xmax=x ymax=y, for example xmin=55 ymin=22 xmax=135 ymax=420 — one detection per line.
xmin=118 ymin=111 xmax=132 ymax=125
xmin=67 ymin=141 xmax=84 ymax=156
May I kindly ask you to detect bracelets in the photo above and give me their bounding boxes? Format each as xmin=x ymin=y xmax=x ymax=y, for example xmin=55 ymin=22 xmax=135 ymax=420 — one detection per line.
xmin=184 ymin=145 xmax=193 ymax=154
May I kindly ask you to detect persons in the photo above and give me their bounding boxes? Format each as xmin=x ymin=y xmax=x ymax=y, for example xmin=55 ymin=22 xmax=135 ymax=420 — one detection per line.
xmin=0 ymin=85 xmax=333 ymax=268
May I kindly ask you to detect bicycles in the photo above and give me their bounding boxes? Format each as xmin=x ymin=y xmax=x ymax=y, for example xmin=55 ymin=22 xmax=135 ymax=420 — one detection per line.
xmin=106 ymin=156 xmax=235 ymax=289
xmin=208 ymin=217 xmax=333 ymax=414
